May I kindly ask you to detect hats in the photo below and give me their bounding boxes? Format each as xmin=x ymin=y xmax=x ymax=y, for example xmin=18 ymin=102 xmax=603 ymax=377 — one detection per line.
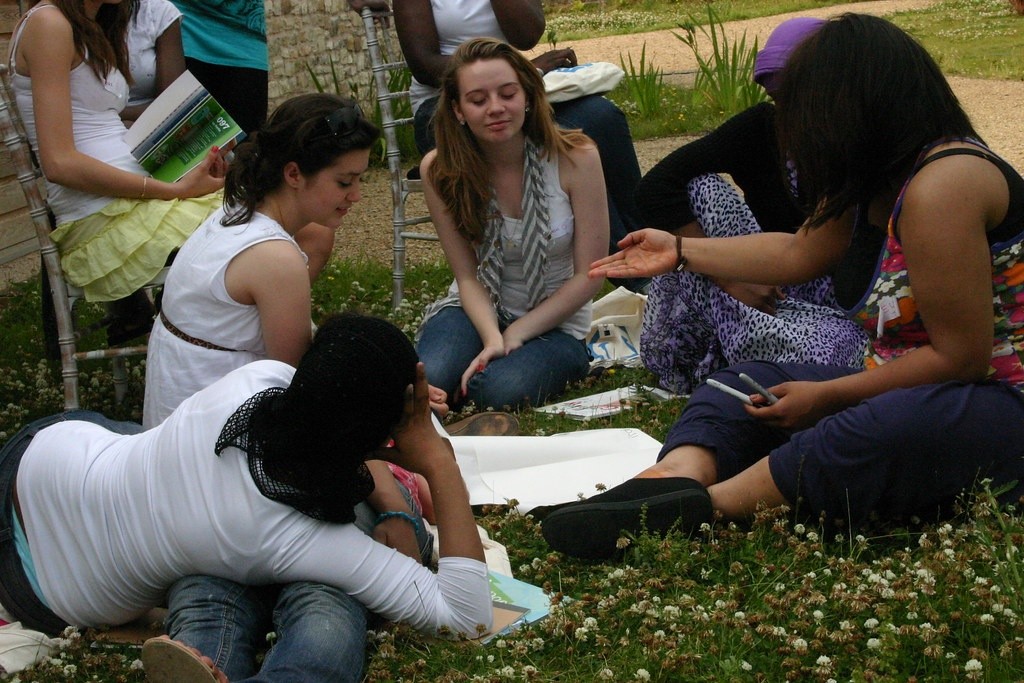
xmin=753 ymin=16 xmax=837 ymax=85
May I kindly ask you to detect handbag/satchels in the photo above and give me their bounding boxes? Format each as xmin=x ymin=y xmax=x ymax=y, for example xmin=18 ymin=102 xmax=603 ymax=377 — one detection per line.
xmin=543 ymin=60 xmax=625 ymax=103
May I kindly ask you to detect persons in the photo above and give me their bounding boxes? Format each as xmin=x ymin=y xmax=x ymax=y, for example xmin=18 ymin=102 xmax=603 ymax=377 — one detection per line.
xmin=0 ymin=0 xmax=1023 ymax=683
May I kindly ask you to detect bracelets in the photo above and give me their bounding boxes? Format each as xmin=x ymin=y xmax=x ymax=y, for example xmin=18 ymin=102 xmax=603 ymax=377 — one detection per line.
xmin=676 ymin=236 xmax=686 ymax=271
xmin=375 ymin=511 xmax=419 ymax=536
xmin=142 ymin=175 xmax=152 ymax=197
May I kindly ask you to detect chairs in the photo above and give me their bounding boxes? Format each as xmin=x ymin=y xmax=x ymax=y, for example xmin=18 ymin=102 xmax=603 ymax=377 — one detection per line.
xmin=362 ymin=5 xmax=441 ymax=312
xmin=0 ymin=63 xmax=173 ymax=413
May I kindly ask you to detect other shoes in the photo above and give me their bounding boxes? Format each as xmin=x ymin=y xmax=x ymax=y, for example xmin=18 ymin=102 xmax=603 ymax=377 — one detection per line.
xmin=541 ymin=477 xmax=714 ymax=562
xmin=140 ymin=637 xmax=230 ymax=683
xmin=444 ymin=411 xmax=520 ymax=437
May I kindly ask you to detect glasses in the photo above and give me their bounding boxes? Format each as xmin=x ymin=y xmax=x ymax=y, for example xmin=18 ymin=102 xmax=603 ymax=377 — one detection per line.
xmin=302 ymin=103 xmax=365 ymax=143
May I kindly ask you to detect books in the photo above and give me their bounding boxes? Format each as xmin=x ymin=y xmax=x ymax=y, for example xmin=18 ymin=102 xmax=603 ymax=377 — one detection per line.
xmin=536 ymin=383 xmax=689 ymax=420
xmin=123 ymin=69 xmax=246 ymax=184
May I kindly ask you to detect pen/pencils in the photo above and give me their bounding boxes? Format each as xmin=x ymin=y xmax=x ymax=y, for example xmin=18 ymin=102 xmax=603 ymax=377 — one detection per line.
xmin=706 ymin=379 xmax=765 ymax=408
xmin=739 ymin=373 xmax=779 ymax=404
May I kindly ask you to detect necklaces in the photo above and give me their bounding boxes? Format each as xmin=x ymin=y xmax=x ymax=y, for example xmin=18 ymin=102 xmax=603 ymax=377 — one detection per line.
xmin=499 ymin=208 xmax=521 ymax=249
